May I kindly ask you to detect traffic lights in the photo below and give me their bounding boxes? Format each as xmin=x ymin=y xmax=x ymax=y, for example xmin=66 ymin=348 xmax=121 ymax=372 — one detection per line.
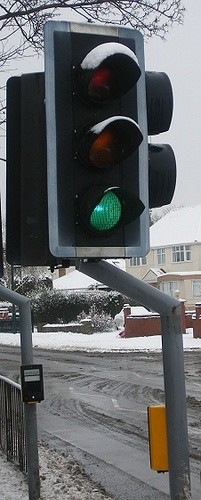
xmin=145 ymin=70 xmax=177 ymax=209
xmin=45 ymin=22 xmax=151 ymax=260
